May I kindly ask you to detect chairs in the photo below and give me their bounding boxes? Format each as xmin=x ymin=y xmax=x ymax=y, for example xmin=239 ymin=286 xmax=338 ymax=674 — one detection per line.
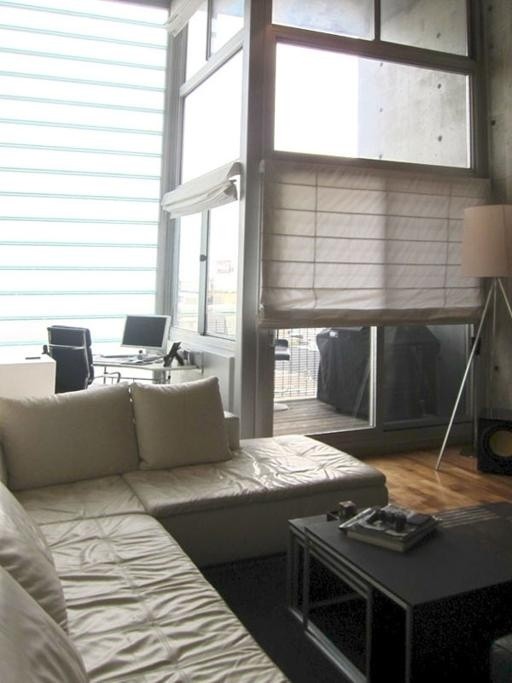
xmin=47 ymin=325 xmax=121 ymax=395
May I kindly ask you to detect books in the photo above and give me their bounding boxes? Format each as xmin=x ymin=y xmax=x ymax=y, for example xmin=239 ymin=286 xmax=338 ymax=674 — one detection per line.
xmin=345 ymin=502 xmax=444 ymax=554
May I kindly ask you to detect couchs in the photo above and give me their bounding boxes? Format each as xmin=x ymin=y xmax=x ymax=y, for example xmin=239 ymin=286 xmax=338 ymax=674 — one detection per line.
xmin=0 ymin=376 xmax=388 ymax=683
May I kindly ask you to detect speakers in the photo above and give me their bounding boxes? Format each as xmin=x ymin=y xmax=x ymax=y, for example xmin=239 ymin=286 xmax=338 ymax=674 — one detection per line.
xmin=477 ymin=418 xmax=512 ymax=476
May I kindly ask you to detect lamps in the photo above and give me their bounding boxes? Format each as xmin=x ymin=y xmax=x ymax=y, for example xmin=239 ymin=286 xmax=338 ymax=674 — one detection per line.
xmin=434 ymin=203 xmax=512 ymax=474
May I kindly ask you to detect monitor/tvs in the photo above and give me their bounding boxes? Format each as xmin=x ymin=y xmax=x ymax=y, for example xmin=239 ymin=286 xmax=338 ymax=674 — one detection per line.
xmin=120 ymin=314 xmax=171 ymax=361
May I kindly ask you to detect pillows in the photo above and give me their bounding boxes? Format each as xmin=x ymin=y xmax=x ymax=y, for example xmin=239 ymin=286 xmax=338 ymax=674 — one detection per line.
xmin=0 ymin=381 xmax=137 ymax=490
xmin=130 ymin=378 xmax=233 ymax=470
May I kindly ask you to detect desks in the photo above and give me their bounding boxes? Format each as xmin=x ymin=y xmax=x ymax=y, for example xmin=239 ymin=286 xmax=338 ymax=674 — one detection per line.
xmin=91 ymin=356 xmax=199 ymax=385
